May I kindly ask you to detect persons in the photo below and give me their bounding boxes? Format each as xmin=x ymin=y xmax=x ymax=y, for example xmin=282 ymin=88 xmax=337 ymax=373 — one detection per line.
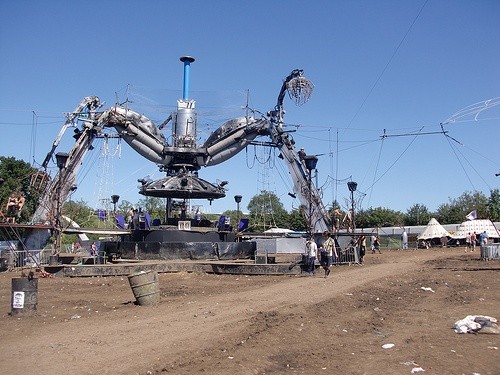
xmin=349 ymin=233 xmax=382 ymax=264
xmin=298 ymin=147 xmax=309 ymax=172
xmin=304 ymin=236 xmax=318 ymax=274
xmin=343 ymin=209 xmax=354 ymax=233
xmin=416 ymin=231 xmax=490 ymax=254
xmin=170 ymin=200 xmax=187 ymax=220
xmin=73 ymin=239 xmax=98 ymax=256
xmin=331 ymin=207 xmax=341 ymax=232
xmin=318 ymin=231 xmax=339 ymax=277
xmin=0 ymin=191 xmax=26 ymax=224
xmin=128 ymin=207 xmax=136 ymax=229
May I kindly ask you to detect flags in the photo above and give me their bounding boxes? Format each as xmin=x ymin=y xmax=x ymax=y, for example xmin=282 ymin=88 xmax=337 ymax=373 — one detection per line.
xmin=465 ymin=210 xmax=477 ymax=220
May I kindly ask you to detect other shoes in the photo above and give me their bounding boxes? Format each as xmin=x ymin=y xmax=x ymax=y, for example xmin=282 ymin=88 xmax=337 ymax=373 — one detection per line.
xmin=326 ymin=269 xmax=330 ymax=276
xmin=324 ymin=276 xmax=327 ymax=278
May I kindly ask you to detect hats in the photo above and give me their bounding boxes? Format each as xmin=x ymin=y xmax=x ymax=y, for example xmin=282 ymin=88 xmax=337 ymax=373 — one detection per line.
xmin=322 ymin=231 xmax=331 ymax=237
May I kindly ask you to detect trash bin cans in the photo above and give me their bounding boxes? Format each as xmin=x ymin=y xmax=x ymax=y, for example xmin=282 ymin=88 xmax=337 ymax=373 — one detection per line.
xmin=480 ymin=243 xmax=500 ymax=260
